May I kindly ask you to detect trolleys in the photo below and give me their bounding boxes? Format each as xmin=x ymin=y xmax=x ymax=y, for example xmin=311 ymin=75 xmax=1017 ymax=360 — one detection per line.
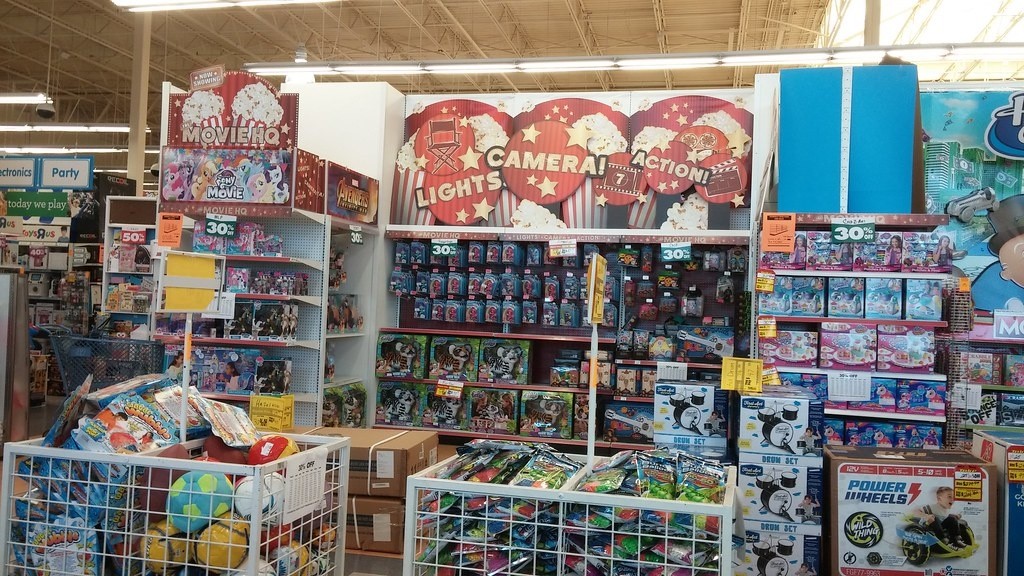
xmin=47 ymin=332 xmax=165 ymax=399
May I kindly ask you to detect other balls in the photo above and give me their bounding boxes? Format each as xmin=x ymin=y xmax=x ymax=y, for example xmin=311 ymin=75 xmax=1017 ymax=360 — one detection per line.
xmin=138 ymin=435 xmax=337 ymax=576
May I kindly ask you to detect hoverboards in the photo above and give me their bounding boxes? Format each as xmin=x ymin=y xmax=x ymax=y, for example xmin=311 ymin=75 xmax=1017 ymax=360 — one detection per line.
xmin=895 ymin=511 xmax=980 ymax=566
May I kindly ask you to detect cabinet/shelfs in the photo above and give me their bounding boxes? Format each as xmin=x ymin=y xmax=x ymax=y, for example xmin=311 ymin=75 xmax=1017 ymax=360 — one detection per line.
xmin=950 ymin=336 xmax=1024 ymax=432
xmin=0 ymin=195 xmax=754 ymax=461
xmin=751 ymin=214 xmax=950 ymax=423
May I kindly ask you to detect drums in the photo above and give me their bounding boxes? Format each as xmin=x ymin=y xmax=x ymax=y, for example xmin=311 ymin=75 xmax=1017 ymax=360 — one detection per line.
xmin=795 ymin=507 xmax=805 ymax=517
xmin=669 ymin=390 xmax=706 ymax=429
xmin=757 ymin=404 xmax=798 ymax=447
xmin=752 ymin=539 xmax=794 ymax=576
xmin=755 ymin=472 xmax=797 ymax=514
xmin=704 ymin=422 xmax=712 ymax=430
xmin=718 ymin=421 xmax=728 ymax=431
xmin=797 ymin=440 xmax=806 ymax=449
xmin=814 ymin=438 xmax=823 ymax=450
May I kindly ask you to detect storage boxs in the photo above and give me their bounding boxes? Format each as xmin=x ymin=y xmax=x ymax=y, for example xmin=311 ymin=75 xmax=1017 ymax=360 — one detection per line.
xmin=318 ymin=159 xmax=379 ymax=226
xmin=0 ymin=228 xmax=1024 ymax=576
xmin=288 ymin=148 xmax=318 ymax=212
xmin=68 ymin=173 xmax=136 ymax=244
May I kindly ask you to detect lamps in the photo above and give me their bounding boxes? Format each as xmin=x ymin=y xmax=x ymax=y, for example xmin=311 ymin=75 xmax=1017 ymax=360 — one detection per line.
xmin=34 ymin=0 xmax=58 ymax=119
xmin=294 ymin=42 xmax=306 ymax=61
xmin=150 ymin=162 xmax=159 ymax=177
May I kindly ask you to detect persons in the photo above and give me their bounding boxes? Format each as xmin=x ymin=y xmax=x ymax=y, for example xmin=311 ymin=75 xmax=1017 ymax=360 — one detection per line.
xmin=139 ymin=432 xmax=154 ymax=444
xmin=885 ymin=235 xmax=902 ymax=265
xmin=168 ymin=351 xmax=184 ymax=378
xmin=933 ymin=235 xmax=952 ymax=266
xmin=58 ymin=226 xmax=69 ymax=242
xmin=864 ymin=340 xmax=877 ymax=351
xmin=924 ymin=281 xmax=942 ymax=297
xmin=802 ymin=427 xmax=822 ymax=456
xmin=801 ymin=494 xmax=820 ymax=518
xmin=222 ymin=362 xmax=241 ymax=389
xmin=795 ymin=563 xmax=817 ymax=576
xmin=709 ymin=411 xmax=726 ymax=437
xmin=806 ymin=337 xmax=817 ymax=349
xmin=922 ymin=342 xmax=935 ymax=354
xmin=69 ymin=192 xmax=81 ymax=217
xmin=851 ymin=278 xmax=864 ymax=291
xmin=93 ymin=407 xmax=128 ymax=432
xmin=913 ymin=486 xmax=967 ymax=551
xmin=789 ymin=234 xmax=807 ymax=263
xmin=812 ymin=277 xmax=823 ymax=289
